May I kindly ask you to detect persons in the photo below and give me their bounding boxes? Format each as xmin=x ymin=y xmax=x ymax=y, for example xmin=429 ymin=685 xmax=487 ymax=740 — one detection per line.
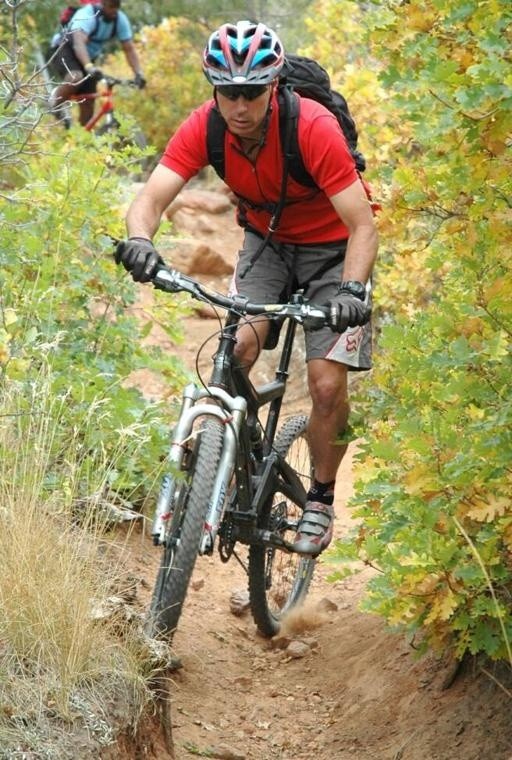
xmin=118 ymin=18 xmax=382 ymax=557
xmin=46 ymin=0 xmax=148 ymax=128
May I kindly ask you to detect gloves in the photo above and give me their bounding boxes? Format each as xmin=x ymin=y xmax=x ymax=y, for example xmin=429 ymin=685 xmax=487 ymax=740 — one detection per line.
xmin=135 ymin=74 xmax=145 ymax=89
xmin=87 ymin=65 xmax=103 ymax=80
xmin=115 ymin=237 xmax=165 ymax=282
xmin=320 ymin=281 xmax=371 ymax=333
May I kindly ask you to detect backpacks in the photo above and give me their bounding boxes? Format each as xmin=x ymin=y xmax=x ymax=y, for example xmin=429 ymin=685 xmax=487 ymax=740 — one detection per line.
xmin=207 ymin=55 xmax=365 ymax=188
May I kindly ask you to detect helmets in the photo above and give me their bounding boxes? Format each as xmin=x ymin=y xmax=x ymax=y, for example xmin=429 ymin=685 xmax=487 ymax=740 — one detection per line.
xmin=201 ymin=21 xmax=285 ymax=86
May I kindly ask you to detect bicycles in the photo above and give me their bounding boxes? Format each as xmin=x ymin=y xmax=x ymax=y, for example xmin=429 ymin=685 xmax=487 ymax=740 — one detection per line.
xmin=39 ymin=67 xmax=149 ymax=188
xmin=83 ymin=230 xmax=373 ymax=661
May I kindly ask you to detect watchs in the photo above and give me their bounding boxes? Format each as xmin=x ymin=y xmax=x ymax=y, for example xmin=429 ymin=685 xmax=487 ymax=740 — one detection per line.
xmin=339 ymin=279 xmax=367 ymax=302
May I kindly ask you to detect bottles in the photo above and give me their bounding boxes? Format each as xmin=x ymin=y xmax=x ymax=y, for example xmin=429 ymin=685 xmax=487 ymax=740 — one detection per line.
xmin=97 ymin=78 xmax=109 ymax=105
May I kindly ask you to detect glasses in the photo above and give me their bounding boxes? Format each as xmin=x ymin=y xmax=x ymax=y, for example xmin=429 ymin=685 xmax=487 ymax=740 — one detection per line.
xmin=217 ymin=85 xmax=267 ymax=101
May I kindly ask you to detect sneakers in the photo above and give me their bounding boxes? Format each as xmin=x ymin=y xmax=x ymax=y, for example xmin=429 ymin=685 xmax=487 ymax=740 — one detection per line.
xmin=294 ymin=500 xmax=334 ymax=553
